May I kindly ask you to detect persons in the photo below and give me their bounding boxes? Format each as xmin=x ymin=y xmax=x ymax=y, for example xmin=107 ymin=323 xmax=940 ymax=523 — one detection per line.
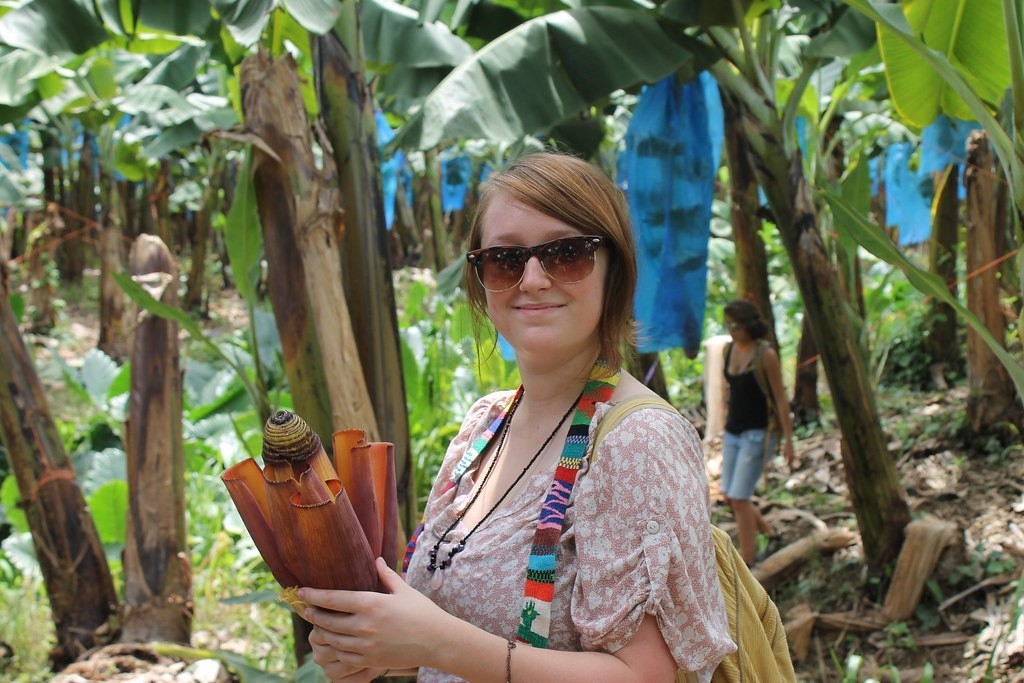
xmin=720 ymin=300 xmax=795 ymax=564
xmin=290 ymin=153 xmax=740 ymax=683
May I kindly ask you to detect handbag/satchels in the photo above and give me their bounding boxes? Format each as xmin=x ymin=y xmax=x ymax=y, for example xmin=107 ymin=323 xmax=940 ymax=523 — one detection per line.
xmin=591 ymin=395 xmax=796 ymax=682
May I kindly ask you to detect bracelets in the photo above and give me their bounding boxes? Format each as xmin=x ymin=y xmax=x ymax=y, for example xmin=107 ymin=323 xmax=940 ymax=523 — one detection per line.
xmin=380 ymin=668 xmax=390 ymax=679
xmin=506 ymin=639 xmax=517 ymax=683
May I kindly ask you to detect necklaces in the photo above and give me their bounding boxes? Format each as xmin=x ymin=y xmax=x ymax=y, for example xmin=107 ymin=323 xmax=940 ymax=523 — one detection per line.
xmin=427 ymin=382 xmax=585 ymax=591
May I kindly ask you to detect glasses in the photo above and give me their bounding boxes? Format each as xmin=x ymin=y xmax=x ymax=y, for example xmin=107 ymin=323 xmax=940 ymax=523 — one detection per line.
xmin=467 ymin=235 xmax=603 ymax=293
xmin=724 ymin=320 xmax=745 ymax=330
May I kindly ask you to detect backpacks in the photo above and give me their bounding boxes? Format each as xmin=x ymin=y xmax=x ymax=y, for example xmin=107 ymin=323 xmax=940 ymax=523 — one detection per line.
xmin=723 ymin=339 xmax=795 ymax=435
